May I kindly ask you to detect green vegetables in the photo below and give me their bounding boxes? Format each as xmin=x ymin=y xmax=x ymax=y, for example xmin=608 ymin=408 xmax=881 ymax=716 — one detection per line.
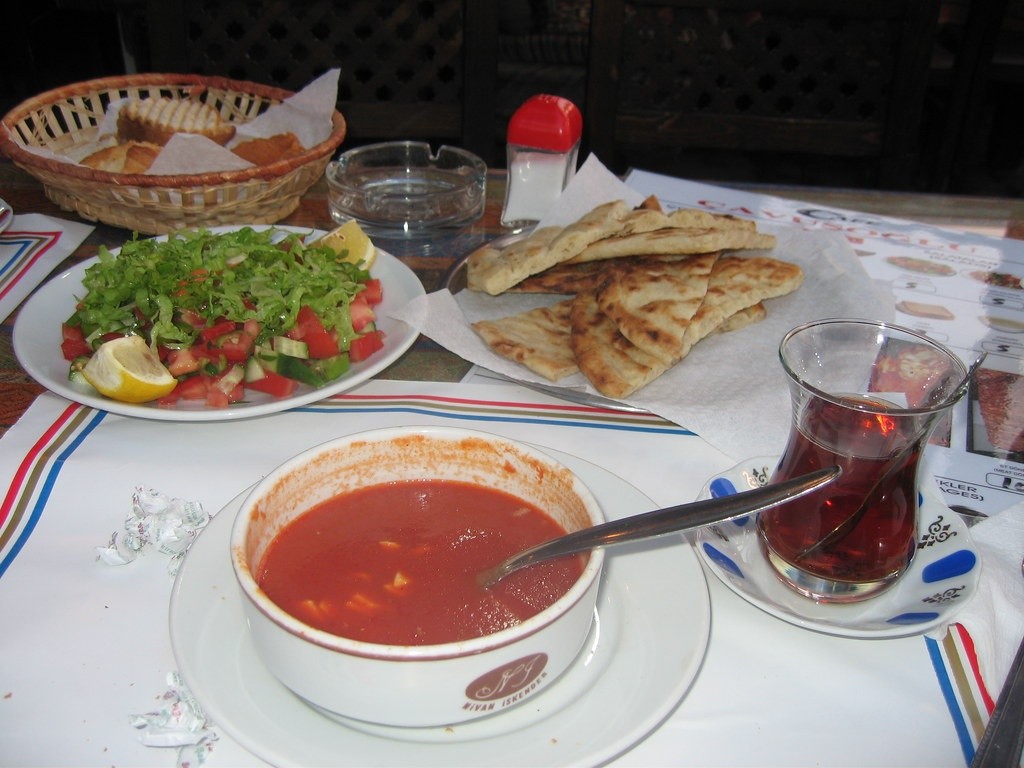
xmin=66 ymin=229 xmax=371 ymax=389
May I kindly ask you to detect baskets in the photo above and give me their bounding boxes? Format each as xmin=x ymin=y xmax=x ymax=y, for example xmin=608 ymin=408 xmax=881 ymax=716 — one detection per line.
xmin=0 ymin=73 xmax=347 ymax=236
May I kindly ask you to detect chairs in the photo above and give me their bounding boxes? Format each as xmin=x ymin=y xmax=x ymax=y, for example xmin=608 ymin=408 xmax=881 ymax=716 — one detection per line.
xmin=588 ymin=0 xmax=941 ymax=193
xmin=145 ymin=1 xmax=500 ymax=167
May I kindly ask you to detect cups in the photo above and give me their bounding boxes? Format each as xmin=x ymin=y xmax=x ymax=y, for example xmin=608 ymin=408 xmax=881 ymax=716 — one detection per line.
xmin=753 ymin=319 xmax=972 ymax=604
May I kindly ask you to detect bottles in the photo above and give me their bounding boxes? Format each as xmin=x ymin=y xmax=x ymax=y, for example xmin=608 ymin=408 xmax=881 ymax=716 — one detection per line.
xmin=500 ymin=93 xmax=583 ymax=230
xmin=964 ymin=269 xmax=1024 ymax=295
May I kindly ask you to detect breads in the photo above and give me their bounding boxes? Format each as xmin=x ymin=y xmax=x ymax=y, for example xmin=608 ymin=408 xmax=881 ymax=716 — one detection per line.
xmin=79 ymin=97 xmax=305 ymax=172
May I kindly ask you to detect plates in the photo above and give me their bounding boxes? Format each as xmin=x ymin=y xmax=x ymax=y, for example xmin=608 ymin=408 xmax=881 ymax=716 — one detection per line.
xmin=694 ymin=453 xmax=980 ymax=641
xmin=979 ymin=315 xmax=1024 ymax=333
xmin=171 ymin=424 xmax=711 ymax=768
xmin=886 ymin=255 xmax=956 ymax=280
xmin=11 ymin=223 xmax=426 ymax=421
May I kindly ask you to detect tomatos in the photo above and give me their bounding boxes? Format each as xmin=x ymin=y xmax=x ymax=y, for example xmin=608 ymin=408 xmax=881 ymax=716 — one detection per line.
xmin=60 ymin=268 xmax=386 ymax=412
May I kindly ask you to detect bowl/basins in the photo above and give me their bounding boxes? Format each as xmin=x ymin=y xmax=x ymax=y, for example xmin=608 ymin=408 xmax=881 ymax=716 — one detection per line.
xmin=229 ymin=440 xmax=608 ymax=726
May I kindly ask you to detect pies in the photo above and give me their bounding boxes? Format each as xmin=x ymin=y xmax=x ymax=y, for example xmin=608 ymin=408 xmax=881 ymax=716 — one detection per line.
xmin=468 ymin=198 xmax=802 ymax=396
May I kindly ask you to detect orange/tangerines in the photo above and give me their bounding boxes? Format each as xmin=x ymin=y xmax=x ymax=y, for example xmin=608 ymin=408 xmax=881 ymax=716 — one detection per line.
xmin=309 ymin=219 xmax=375 ymax=278
xmin=82 ymin=335 xmax=177 ymax=403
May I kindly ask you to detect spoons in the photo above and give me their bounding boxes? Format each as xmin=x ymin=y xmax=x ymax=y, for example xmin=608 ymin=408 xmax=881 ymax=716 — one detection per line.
xmin=475 ymin=463 xmax=843 ymax=595
xmin=795 ymin=350 xmax=989 ymax=565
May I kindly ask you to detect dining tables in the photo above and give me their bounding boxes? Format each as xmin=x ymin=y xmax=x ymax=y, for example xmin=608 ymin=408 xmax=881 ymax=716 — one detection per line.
xmin=0 ymin=156 xmax=1023 ymax=768
